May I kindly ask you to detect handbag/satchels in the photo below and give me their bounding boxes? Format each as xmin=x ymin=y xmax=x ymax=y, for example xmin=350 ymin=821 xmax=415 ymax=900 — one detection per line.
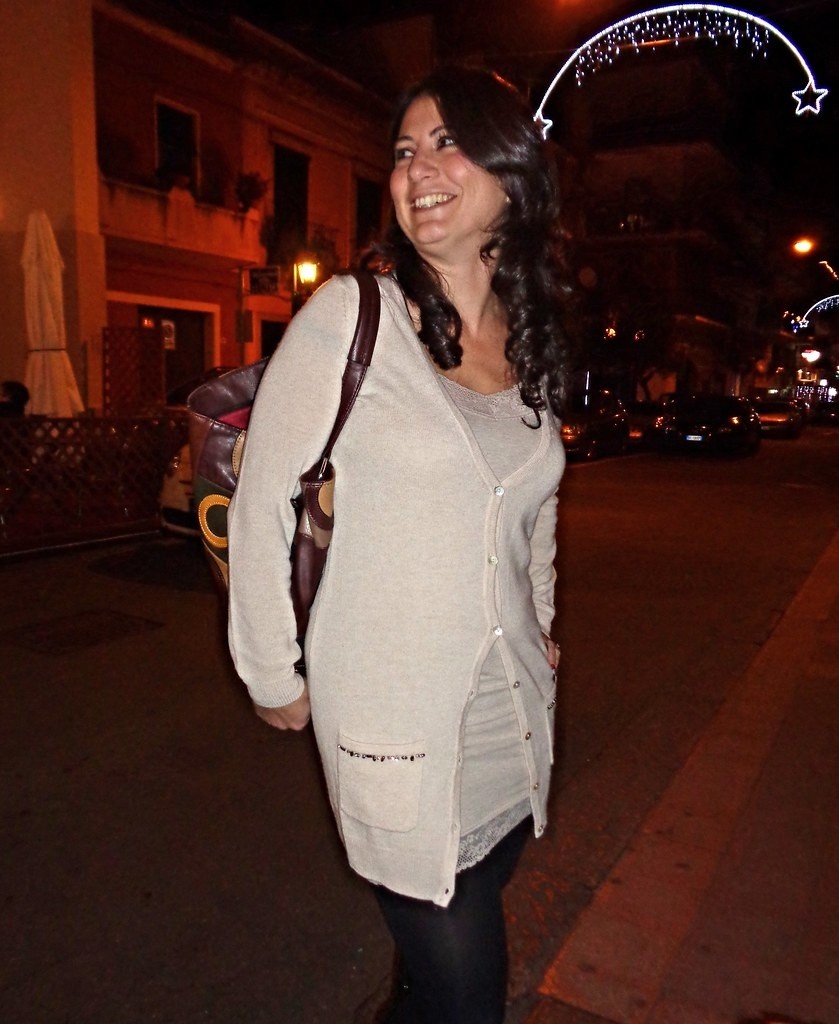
xmin=187 ymin=270 xmax=382 ymax=619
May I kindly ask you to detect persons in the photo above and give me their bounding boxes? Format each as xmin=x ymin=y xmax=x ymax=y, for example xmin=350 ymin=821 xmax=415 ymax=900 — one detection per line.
xmin=227 ymin=72 xmax=567 ymax=1024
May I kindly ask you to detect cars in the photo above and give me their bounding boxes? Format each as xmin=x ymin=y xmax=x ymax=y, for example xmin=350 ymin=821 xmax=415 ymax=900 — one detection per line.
xmin=746 ymin=401 xmax=802 ymax=439
xmin=656 ymin=391 xmax=760 ymax=458
xmin=156 ymin=444 xmax=203 ymax=539
xmin=557 ymin=390 xmax=646 ymax=462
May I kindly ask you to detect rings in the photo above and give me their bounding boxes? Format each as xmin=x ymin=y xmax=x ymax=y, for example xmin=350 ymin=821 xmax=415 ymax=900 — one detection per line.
xmin=555 ymin=644 xmax=560 ymax=649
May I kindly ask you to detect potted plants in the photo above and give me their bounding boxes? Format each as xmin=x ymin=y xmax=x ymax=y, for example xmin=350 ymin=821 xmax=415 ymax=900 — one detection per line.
xmin=234 ymin=173 xmax=268 ymax=213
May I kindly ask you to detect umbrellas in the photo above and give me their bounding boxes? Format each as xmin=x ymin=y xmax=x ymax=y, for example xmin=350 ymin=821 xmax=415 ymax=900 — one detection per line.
xmin=20 ymin=206 xmax=85 ymax=466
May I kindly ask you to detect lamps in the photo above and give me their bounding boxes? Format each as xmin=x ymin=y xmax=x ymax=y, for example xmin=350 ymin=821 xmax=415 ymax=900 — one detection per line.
xmin=292 ymin=249 xmax=320 ymax=283
